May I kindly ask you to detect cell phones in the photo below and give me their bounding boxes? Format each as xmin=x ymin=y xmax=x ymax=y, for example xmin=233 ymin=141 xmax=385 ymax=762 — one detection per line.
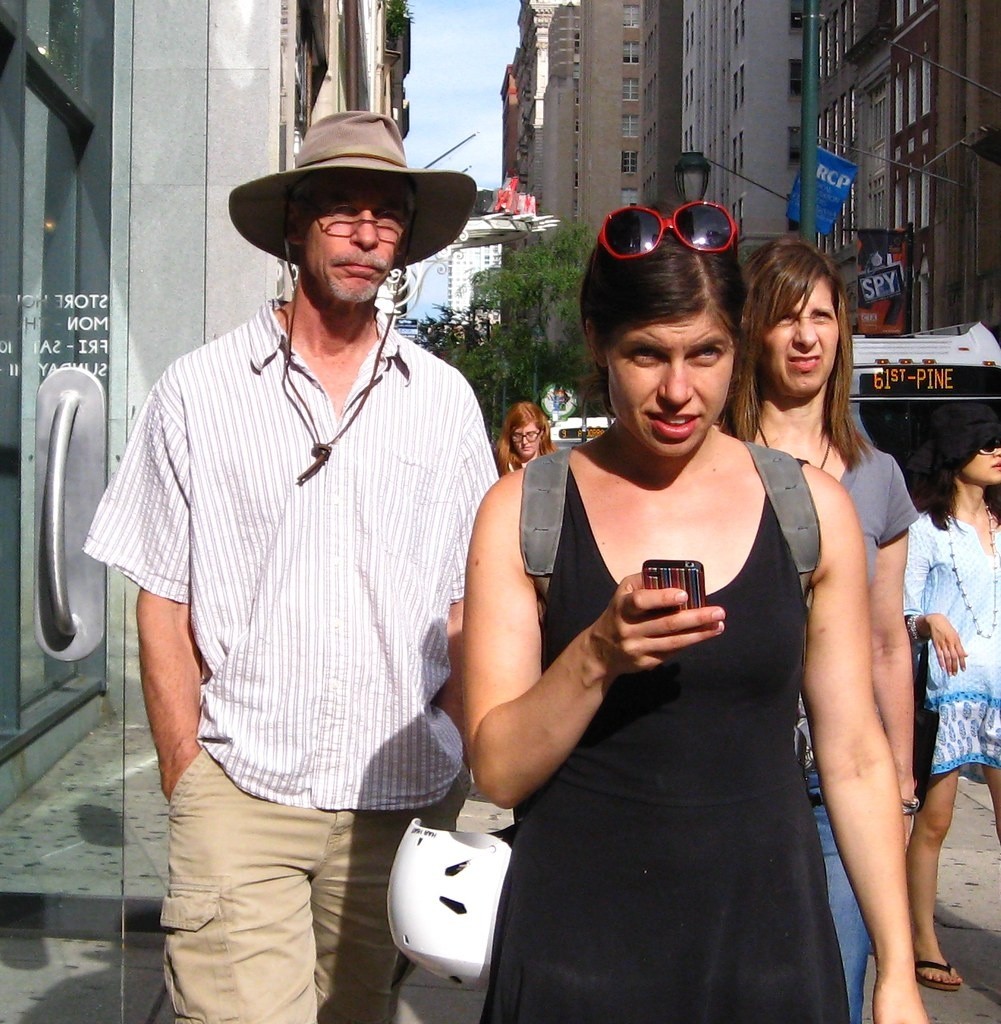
xmin=642 ymin=560 xmax=706 ymax=616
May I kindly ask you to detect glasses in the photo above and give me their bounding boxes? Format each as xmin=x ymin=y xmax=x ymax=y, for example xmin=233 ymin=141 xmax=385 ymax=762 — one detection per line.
xmin=512 ymin=430 xmax=542 ymax=443
xmin=598 ymin=200 xmax=738 ymax=259
xmin=299 ymin=194 xmax=409 ymax=230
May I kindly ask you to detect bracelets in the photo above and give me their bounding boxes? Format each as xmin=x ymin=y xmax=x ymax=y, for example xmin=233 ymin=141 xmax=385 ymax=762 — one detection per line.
xmin=907 ymin=614 xmax=930 ymax=643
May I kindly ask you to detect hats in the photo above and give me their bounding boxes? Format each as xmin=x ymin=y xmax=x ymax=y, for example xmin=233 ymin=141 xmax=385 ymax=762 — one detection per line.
xmin=229 ymin=111 xmax=476 ymax=267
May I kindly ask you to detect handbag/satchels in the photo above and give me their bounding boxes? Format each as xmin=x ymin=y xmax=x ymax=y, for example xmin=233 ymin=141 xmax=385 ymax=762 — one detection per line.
xmin=905 ymin=613 xmax=940 ymax=815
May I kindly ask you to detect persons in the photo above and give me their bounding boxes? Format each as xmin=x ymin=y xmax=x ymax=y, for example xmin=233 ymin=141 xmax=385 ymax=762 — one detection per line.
xmin=461 ymin=200 xmax=928 ymax=1024
xmin=716 ymin=234 xmax=1001 ymax=1024
xmin=84 ymin=112 xmax=503 ymax=1024
xmin=494 ymin=401 xmax=557 ymax=479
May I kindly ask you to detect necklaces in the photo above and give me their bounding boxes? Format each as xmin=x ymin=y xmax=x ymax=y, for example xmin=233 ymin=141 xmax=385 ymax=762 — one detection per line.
xmin=758 ymin=424 xmax=833 ymax=470
xmin=944 ymin=505 xmax=997 ymax=638
